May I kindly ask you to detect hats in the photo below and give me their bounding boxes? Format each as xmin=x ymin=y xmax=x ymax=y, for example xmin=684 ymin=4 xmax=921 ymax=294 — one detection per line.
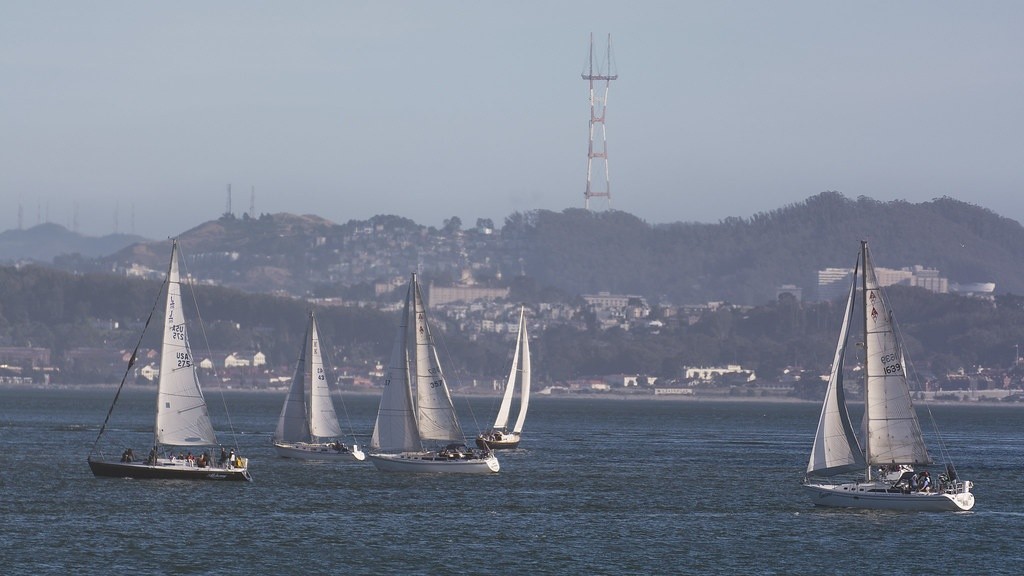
xmin=231 ymin=451 xmax=234 ymax=453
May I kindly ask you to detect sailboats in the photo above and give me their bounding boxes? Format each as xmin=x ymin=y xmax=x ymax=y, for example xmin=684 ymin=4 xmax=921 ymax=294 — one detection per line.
xmin=476 ymin=304 xmax=531 ymax=451
xmin=273 ymin=308 xmax=363 ymax=462
xmin=801 ymin=239 xmax=974 ymax=514
xmin=88 ymin=240 xmax=251 ymax=480
xmin=366 ymin=273 xmax=501 ymax=475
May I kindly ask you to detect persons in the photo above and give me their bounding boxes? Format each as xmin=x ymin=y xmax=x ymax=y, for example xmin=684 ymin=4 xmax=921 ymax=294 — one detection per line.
xmin=120 ymin=446 xmax=158 ymax=466
xmin=918 ymin=472 xmax=931 ymax=492
xmin=168 ymin=446 xmax=244 ymax=471
